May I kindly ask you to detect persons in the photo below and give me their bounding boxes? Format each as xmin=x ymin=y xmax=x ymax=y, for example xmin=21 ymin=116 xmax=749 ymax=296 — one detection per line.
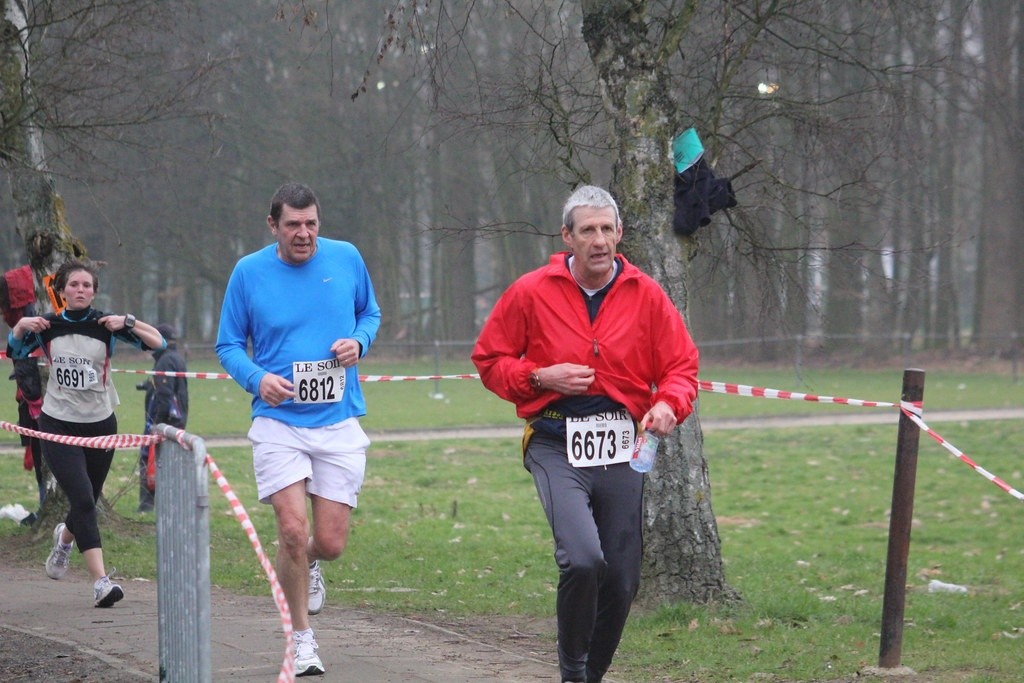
xmin=471 ymin=183 xmax=700 ymax=683
xmin=136 ymin=324 xmax=190 ymax=507
xmin=6 ymin=260 xmax=169 ymax=608
xmin=215 ymin=183 xmax=382 ymax=676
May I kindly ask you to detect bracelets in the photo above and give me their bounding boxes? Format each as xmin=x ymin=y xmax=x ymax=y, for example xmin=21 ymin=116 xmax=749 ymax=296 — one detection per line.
xmin=536 ymin=376 xmax=541 ymax=388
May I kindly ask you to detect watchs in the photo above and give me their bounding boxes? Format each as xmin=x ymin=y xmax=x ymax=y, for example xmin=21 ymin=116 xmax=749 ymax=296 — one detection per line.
xmin=123 ymin=313 xmax=135 ymax=332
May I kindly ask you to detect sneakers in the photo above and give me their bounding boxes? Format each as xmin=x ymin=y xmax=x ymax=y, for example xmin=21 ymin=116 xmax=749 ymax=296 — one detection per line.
xmin=292 ymin=630 xmax=325 ymax=676
xmin=45 ymin=523 xmax=73 ymax=579
xmin=305 ymin=560 xmax=327 ymax=615
xmin=92 ymin=567 xmax=124 ymax=608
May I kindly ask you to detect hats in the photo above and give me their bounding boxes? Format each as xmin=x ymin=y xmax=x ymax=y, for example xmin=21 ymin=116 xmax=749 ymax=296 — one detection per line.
xmin=140 ymin=323 xmax=178 ymax=349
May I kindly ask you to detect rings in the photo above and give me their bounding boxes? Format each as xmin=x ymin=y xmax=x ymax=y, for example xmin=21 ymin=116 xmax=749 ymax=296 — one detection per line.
xmin=345 ymin=359 xmax=348 ymax=365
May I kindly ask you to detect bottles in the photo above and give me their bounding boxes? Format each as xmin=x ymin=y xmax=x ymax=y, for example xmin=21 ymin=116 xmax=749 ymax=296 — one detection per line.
xmin=630 ymin=422 xmax=661 ymax=472
